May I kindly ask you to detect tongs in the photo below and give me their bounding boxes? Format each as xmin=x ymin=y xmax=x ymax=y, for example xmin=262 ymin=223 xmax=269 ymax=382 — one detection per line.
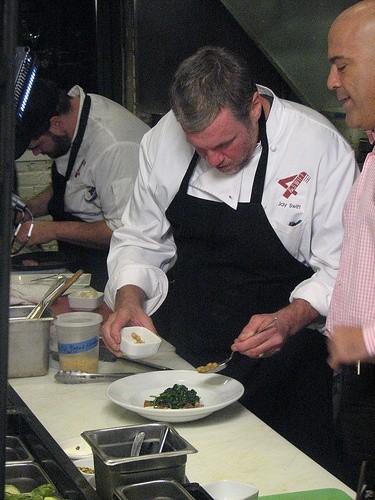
xmin=54 ymin=346 xmax=172 ymax=384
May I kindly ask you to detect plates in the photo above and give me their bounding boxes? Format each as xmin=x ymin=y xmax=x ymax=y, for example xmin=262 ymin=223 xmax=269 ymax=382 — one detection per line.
xmin=72 ymin=459 xmax=95 ymax=476
xmin=59 ymin=437 xmax=94 ymax=459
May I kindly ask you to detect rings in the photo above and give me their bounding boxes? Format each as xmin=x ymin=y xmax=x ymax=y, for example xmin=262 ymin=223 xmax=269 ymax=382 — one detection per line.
xmin=259 ymin=353 xmax=268 ymax=358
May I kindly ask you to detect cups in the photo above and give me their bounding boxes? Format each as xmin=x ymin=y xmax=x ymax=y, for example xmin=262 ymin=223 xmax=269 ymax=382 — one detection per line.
xmin=53 ymin=312 xmax=103 ymax=374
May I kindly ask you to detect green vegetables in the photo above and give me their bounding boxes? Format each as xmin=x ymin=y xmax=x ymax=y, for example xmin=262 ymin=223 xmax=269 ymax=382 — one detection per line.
xmin=150 ymin=383 xmax=199 ymax=409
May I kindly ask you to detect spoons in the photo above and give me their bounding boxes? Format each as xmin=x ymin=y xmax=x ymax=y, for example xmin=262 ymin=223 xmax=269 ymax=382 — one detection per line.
xmin=197 ymin=317 xmax=278 ymax=374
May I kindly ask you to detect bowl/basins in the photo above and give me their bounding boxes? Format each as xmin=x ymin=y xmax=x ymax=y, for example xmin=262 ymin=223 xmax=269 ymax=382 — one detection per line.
xmin=202 ymin=480 xmax=258 ymax=500
xmin=119 ymin=326 xmax=161 ymax=359
xmin=68 ymin=291 xmax=104 ymax=311
xmin=107 ymin=369 xmax=245 ymax=422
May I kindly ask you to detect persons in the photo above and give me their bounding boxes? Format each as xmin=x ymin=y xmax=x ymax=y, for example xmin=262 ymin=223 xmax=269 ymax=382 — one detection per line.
xmin=102 ymin=47 xmax=360 ymax=468
xmin=327 ymin=0 xmax=375 ymax=500
xmin=11 ymin=76 xmax=151 ymax=293
